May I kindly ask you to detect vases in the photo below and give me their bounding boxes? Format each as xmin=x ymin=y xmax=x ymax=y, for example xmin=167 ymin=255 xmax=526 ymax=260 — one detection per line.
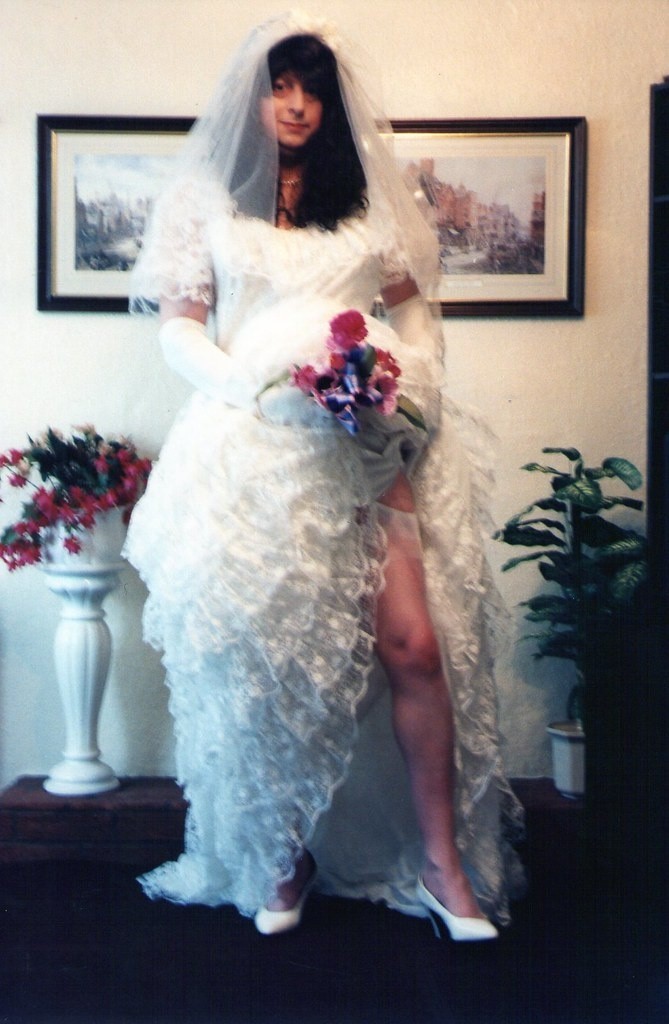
xmin=49 ymin=504 xmax=128 ymax=576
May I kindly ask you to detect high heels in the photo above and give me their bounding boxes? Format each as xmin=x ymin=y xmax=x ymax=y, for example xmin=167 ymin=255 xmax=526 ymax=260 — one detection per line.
xmin=416 ymin=872 xmax=499 ymax=942
xmin=255 ymin=848 xmax=319 ymax=934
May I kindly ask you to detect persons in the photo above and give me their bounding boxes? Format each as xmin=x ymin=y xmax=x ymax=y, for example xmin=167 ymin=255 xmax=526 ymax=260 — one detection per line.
xmin=120 ymin=11 xmax=525 ymax=940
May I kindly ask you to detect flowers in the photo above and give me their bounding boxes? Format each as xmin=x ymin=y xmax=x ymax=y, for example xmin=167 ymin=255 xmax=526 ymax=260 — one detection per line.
xmin=296 ymin=307 xmax=402 ymax=428
xmin=0 ymin=423 xmax=152 ymax=570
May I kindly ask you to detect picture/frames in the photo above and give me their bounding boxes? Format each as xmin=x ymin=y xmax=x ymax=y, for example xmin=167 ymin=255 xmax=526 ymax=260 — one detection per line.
xmin=33 ymin=113 xmax=588 ymax=318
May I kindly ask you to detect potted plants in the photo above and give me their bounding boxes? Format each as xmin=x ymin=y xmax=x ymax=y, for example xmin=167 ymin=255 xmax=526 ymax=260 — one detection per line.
xmin=490 ymin=442 xmax=648 ymax=803
